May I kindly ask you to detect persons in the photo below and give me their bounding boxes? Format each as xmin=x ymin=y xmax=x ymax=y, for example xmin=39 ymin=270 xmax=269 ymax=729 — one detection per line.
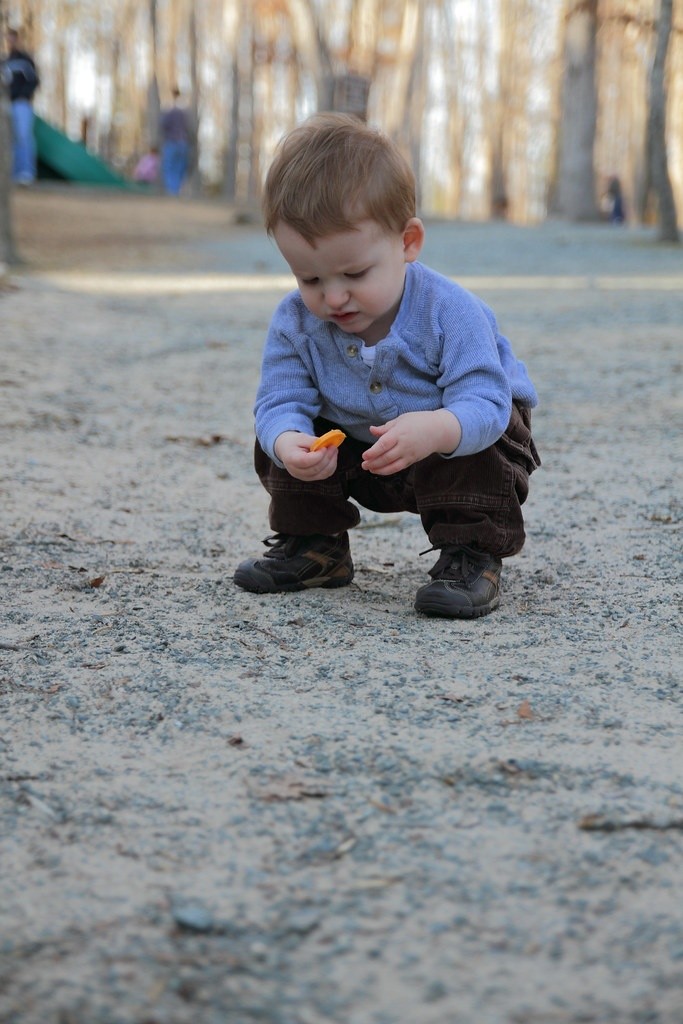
xmin=159 ymin=88 xmax=193 ymax=196
xmin=4 ymin=28 xmax=40 ymax=186
xmin=231 ymin=113 xmax=539 ymax=619
xmin=133 ymin=146 xmax=160 ymax=183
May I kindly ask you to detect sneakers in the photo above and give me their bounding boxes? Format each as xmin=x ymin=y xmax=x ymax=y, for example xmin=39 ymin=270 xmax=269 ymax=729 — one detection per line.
xmin=415 ymin=540 xmax=504 ymax=619
xmin=234 ymin=526 xmax=353 ymax=594
